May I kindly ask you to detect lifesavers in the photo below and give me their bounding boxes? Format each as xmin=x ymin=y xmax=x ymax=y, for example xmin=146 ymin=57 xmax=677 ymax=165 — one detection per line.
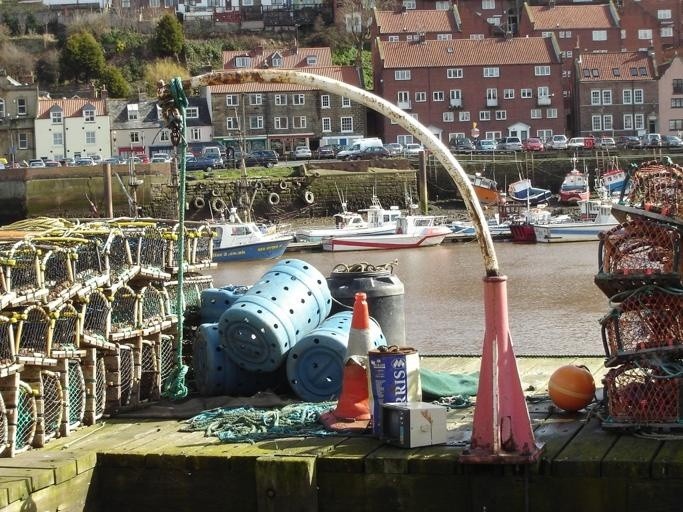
xmin=305 ymin=191 xmax=314 ymax=204
xmin=212 ymin=198 xmax=225 ymax=213
xmin=211 ymin=188 xmax=220 ymax=196
xmin=279 ymin=181 xmax=288 ymax=190
xmin=255 ymin=182 xmax=263 ymax=190
xmin=193 ymin=197 xmax=205 ymax=209
xmin=267 ymin=192 xmax=280 ymax=206
xmin=314 ymin=172 xmax=321 ymax=178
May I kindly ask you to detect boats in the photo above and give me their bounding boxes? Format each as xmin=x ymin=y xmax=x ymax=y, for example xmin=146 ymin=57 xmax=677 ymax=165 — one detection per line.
xmin=288 ymin=193 xmax=454 ymax=251
xmin=213 ymin=220 xmax=296 ymax=261
xmin=445 ymin=149 xmax=632 ymax=241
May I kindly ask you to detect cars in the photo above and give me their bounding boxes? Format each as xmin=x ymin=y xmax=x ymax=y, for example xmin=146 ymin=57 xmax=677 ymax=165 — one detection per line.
xmin=351 ymin=146 xmax=389 ymax=159
xmin=224 ymin=149 xmax=278 ymax=168
xmin=295 ymin=146 xmax=311 ymax=159
xmin=388 ymin=142 xmax=424 ymax=156
xmin=315 ymin=146 xmax=334 ymax=159
xmin=447 ymin=133 xmax=683 ymax=154
xmin=203 ymin=153 xmax=224 ymax=168
xmin=7 ymin=152 xmax=173 ymax=168
xmin=179 ymin=156 xmax=216 ymax=171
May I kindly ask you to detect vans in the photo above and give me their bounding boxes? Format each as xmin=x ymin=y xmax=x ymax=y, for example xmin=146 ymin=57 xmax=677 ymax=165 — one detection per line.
xmin=201 ymin=146 xmax=221 ymax=155
xmin=336 ymin=137 xmax=383 ymax=159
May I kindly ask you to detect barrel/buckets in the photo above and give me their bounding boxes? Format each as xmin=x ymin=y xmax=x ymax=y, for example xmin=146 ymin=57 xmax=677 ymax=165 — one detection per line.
xmin=370 ymin=345 xmax=420 ymax=439
xmin=327 ymin=267 xmax=405 ymax=347
